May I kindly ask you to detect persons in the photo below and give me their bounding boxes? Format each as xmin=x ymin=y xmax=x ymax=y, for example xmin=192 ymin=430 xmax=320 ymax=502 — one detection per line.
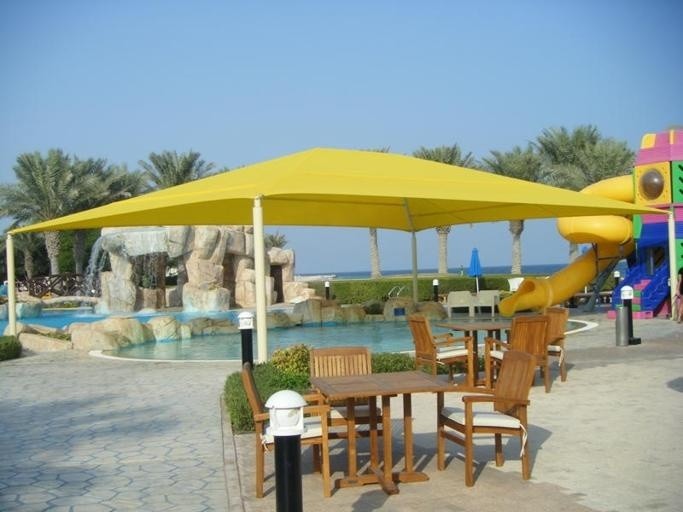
xmin=674 ymin=263 xmax=682 ymax=324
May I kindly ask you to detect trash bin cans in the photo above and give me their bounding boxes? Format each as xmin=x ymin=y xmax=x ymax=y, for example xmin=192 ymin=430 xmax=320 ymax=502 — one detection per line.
xmin=615 ymin=306 xmax=629 ymax=346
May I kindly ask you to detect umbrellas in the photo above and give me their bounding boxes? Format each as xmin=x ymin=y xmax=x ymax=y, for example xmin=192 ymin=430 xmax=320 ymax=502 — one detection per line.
xmin=467 ymin=248 xmax=484 ymax=313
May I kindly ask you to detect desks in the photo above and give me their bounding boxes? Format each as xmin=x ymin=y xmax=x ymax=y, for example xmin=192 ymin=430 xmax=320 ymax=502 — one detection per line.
xmin=437 ymin=320 xmax=513 ymax=393
xmin=308 ymin=369 xmax=454 ymax=496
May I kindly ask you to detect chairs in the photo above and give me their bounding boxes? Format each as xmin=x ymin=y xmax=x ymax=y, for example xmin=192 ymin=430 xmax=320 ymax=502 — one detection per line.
xmin=484 ymin=316 xmax=551 ymax=394
xmin=308 ymin=346 xmax=381 ymax=478
xmin=405 ymin=316 xmax=475 ymax=386
xmin=242 ymin=362 xmax=332 ymax=499
xmin=448 ymin=290 xmax=500 ymax=318
xmin=432 ymin=350 xmax=536 ymax=487
xmin=540 ymin=307 xmax=568 ymax=382
